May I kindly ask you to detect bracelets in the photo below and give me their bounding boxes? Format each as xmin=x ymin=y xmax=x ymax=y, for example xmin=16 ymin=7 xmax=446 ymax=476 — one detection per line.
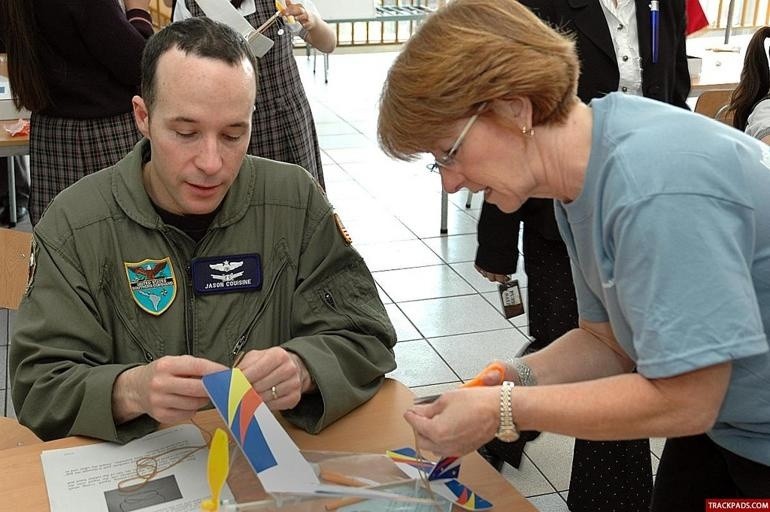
xmin=507 ymin=357 xmax=535 ymax=386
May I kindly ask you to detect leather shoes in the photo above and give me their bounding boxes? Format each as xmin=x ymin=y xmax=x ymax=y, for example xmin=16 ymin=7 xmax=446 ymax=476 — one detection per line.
xmin=0 ymin=207 xmax=27 ymax=224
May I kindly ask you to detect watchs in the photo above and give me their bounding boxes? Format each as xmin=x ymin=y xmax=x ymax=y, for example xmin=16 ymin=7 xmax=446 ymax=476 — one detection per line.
xmin=494 ymin=382 xmax=519 ymax=444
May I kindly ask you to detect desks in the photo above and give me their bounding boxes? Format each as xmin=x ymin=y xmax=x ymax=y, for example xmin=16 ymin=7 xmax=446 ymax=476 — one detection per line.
xmin=1 ymin=377 xmax=539 ymax=509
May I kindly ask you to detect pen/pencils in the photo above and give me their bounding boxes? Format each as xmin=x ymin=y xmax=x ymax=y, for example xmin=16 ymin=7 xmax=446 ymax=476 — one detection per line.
xmin=314 ymin=468 xmax=444 ymax=511
xmin=647 ymin=1 xmax=660 ymax=64
xmin=713 ymin=48 xmax=739 ymax=53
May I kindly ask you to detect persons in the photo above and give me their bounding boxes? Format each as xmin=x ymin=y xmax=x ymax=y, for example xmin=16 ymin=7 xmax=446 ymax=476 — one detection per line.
xmin=0 ymin=0 xmax=394 ymax=445
xmin=376 ymin=0 xmax=770 ymax=512
xmin=724 ymin=25 xmax=770 ymax=143
xmin=473 ymin=0 xmax=689 ymax=512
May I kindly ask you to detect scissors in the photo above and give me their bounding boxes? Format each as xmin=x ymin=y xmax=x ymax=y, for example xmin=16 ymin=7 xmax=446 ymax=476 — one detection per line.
xmin=413 ymin=363 xmax=505 ymax=405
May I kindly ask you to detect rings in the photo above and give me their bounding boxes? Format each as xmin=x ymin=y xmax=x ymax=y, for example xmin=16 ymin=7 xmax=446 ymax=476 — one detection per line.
xmin=479 ymin=270 xmax=485 ymax=274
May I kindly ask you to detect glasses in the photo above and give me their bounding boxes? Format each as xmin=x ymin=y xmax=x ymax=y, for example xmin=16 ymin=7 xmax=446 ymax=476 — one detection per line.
xmin=426 ymin=103 xmax=486 ymax=173
